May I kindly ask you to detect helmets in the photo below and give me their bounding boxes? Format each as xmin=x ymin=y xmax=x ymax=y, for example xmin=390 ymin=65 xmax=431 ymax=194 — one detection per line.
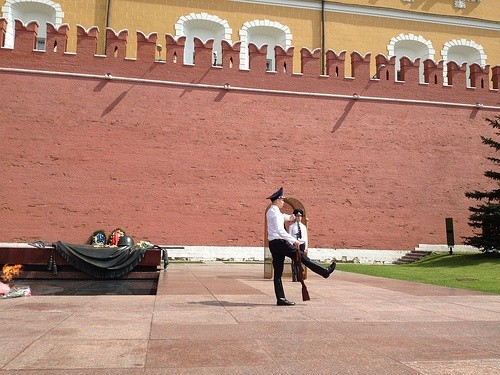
xmin=117 ymin=235 xmax=134 ymax=246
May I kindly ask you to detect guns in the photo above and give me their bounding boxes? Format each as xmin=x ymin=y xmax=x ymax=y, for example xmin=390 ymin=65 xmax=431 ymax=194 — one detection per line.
xmin=294 ymin=232 xmax=311 ymax=302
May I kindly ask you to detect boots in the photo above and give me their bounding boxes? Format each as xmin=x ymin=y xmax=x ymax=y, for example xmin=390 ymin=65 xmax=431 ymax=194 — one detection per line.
xmin=306 ymin=260 xmax=336 ymax=279
xmin=292 ymin=266 xmax=298 ymax=281
xmin=273 ymin=279 xmax=295 ymax=305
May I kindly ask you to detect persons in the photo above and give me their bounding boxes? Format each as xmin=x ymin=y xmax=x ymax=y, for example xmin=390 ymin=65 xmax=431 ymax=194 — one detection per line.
xmin=289 ymin=209 xmax=308 ymax=282
xmin=266 ymin=187 xmax=337 ymax=305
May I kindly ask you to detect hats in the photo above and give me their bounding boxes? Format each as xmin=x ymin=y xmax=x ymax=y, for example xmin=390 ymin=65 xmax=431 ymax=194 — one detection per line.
xmin=266 ymin=187 xmax=286 ymax=201
xmin=292 ymin=209 xmax=303 ymax=218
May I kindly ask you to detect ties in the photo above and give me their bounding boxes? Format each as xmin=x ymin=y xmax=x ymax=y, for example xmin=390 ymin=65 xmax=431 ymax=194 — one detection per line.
xmin=297 ymin=224 xmax=302 ymax=240
